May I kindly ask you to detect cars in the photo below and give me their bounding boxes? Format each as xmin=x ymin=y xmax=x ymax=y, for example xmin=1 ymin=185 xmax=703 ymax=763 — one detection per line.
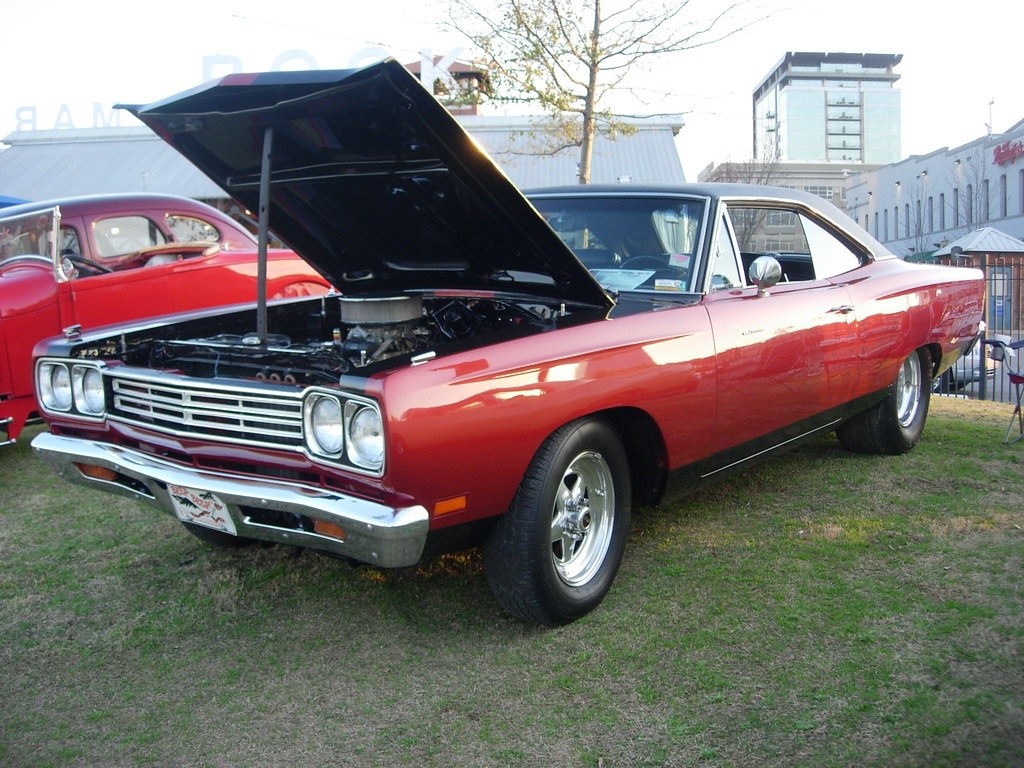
xmin=30 ymin=60 xmax=991 ymax=628
xmin=930 ymin=320 xmax=1000 ymax=393
xmin=0 ymin=191 xmax=341 ymax=448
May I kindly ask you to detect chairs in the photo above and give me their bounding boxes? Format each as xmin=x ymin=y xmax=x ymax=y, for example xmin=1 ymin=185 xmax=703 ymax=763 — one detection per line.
xmin=981 ymin=339 xmax=1024 ymax=445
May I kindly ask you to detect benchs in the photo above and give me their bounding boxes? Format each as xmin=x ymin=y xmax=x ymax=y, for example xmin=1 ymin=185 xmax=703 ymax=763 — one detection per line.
xmin=616 ymin=252 xmax=816 ymax=286
